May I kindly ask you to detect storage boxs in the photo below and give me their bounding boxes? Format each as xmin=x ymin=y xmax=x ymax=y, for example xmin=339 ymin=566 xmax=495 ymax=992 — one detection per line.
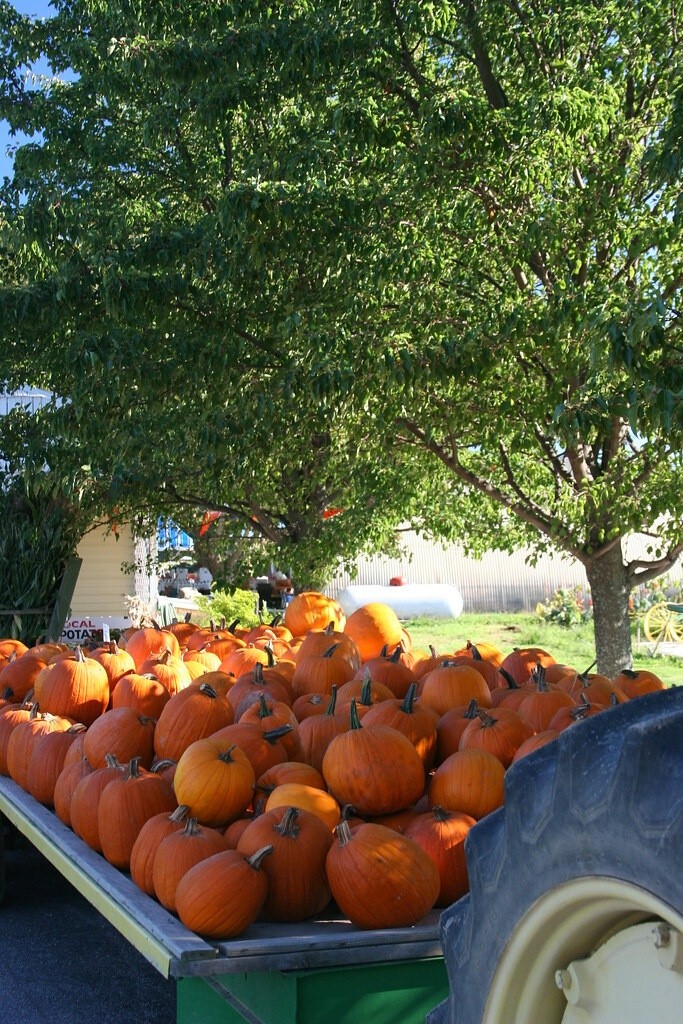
xmin=176 ymin=956 xmax=449 ymax=1024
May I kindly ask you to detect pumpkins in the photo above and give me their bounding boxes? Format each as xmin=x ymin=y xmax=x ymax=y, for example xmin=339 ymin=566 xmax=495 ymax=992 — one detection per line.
xmin=0 ymin=593 xmax=669 ymax=939
